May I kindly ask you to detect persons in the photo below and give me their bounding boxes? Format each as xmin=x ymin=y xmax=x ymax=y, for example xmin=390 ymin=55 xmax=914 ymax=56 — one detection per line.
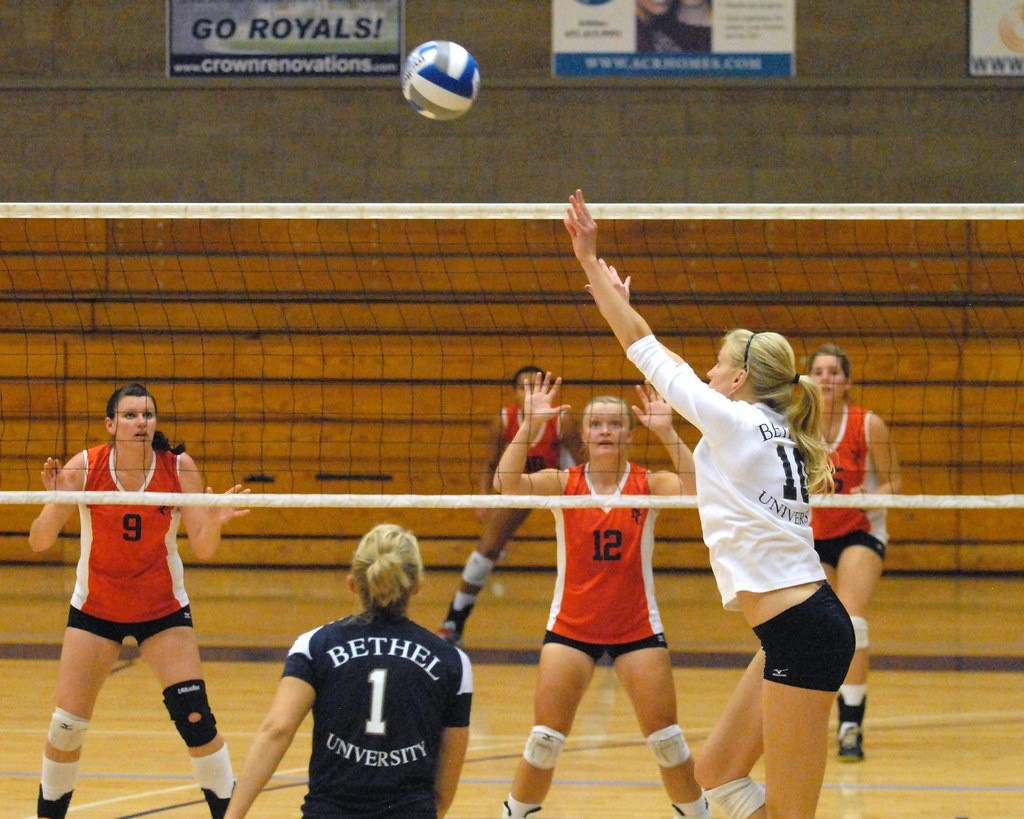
xmin=25 ymin=381 xmax=253 ymax=819
xmin=796 ymin=343 xmax=901 ymax=763
xmin=218 ymin=522 xmax=475 ymax=819
xmin=561 ymin=185 xmax=856 ymax=819
xmin=488 ymin=369 xmax=713 ymax=819
xmin=431 ymin=362 xmax=586 ymax=649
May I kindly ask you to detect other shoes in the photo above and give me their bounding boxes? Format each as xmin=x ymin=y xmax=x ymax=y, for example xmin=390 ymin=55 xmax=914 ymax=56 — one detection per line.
xmin=436 ymin=620 xmax=462 ymax=645
xmin=836 ymin=721 xmax=864 ymax=762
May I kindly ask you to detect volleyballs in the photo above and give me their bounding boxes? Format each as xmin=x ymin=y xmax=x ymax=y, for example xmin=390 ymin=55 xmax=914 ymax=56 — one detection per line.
xmin=399 ymin=39 xmax=482 ymax=122
